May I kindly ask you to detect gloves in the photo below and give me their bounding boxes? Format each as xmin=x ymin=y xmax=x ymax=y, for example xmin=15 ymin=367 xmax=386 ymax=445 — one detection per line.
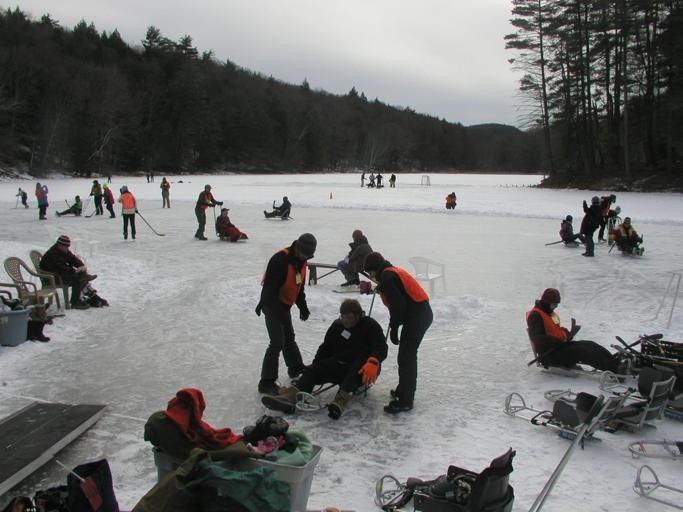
xmin=299 ymin=308 xmax=310 ymax=321
xmin=390 ymin=328 xmax=399 ymax=345
xmin=255 ymin=304 xmax=263 ymax=316
xmin=359 ymin=357 xmax=379 ymax=386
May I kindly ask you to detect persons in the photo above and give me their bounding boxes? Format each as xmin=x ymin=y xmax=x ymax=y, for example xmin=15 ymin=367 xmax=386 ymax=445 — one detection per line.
xmin=450 ymin=191 xmax=457 ymax=209
xmin=215 ymin=208 xmax=248 ymax=240
xmin=15 ymin=187 xmax=29 ymax=208
xmin=193 ymin=184 xmax=223 ymax=241
xmin=445 ymin=194 xmax=453 ymax=209
xmin=253 ymin=233 xmax=316 ymax=396
xmin=389 ymin=173 xmax=396 ymax=188
xmin=56 ymin=194 xmax=83 ymax=217
xmin=365 ymin=250 xmax=435 ymax=412
xmin=376 ymin=173 xmax=384 ymax=189
xmin=90 ymin=179 xmax=103 ymax=215
xmin=107 ymin=173 xmax=112 ymax=183
xmin=263 ymin=196 xmax=292 ymax=217
xmin=337 ymin=230 xmax=374 ymax=286
xmin=152 ymin=386 xmax=234 ymax=482
xmin=98 ymin=181 xmax=116 ymax=218
xmin=559 ymin=193 xmax=646 ymax=258
xmin=262 ymin=298 xmax=388 ymax=421
xmin=524 ymin=286 xmax=632 ymax=380
xmin=145 ymin=172 xmax=150 ymax=183
xmin=150 ymin=171 xmax=154 ymax=183
xmin=360 ymin=172 xmax=368 ymax=187
xmin=117 ymin=184 xmax=138 ymax=239
xmin=159 ymin=177 xmax=171 ymax=209
xmin=34 ymin=182 xmax=49 ymax=220
xmin=369 ymin=172 xmax=376 ymax=187
xmin=39 ymin=235 xmax=97 ymax=309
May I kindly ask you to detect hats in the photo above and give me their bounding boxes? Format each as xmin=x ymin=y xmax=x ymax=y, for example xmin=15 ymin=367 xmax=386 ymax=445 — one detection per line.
xmin=299 ymin=234 xmax=317 ymax=257
xmin=351 ymin=230 xmax=361 ymax=237
xmin=205 ymin=185 xmax=210 ymax=189
xmin=57 ymin=235 xmax=70 ymax=247
xmin=565 ymin=195 xmax=630 ymax=224
xmin=35 ymin=180 xmax=127 ymax=202
xmin=540 ymin=288 xmax=559 ymax=303
xmin=364 ymin=252 xmax=384 ymax=271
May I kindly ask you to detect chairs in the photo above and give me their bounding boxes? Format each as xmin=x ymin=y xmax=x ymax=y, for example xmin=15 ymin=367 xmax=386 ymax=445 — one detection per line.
xmin=4 ymin=256 xmax=61 ymax=308
xmin=407 ymin=256 xmax=447 ymax=301
xmin=527 ymin=328 xmax=576 ymax=370
xmin=0 ymin=283 xmax=45 ymax=321
xmin=613 ymin=375 xmax=677 ymax=429
xmin=414 ymin=446 xmax=515 ymax=512
xmin=30 ymin=250 xmax=73 ymax=309
xmin=541 ymin=387 xmax=635 ymax=437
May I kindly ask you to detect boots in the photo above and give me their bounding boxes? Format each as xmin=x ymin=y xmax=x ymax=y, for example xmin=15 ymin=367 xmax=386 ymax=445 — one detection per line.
xmin=327 ymin=390 xmax=352 ymax=420
xmin=31 ymin=321 xmax=49 ymax=342
xmin=261 ymin=386 xmax=301 ymax=415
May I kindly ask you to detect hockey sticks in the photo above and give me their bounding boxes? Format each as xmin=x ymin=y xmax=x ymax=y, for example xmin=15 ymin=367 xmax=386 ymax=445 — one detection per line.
xmin=138 ymin=211 xmax=165 ymax=236
xmin=84 ymin=199 xmax=104 ymax=218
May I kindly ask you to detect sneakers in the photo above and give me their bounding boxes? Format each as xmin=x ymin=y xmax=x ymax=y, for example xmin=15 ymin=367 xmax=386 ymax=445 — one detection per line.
xmin=616 ymin=359 xmax=630 ymax=375
xmin=257 ymin=381 xmax=280 ymax=395
xmin=287 ymin=365 xmax=307 ymax=378
xmin=86 ymin=297 xmax=101 ymax=307
xmin=382 ymin=402 xmax=412 ymax=413
xmin=71 ymin=301 xmax=90 ymax=309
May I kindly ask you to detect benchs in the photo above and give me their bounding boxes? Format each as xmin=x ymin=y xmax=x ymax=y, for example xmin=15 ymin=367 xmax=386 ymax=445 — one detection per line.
xmin=306 ymin=262 xmax=377 ymax=285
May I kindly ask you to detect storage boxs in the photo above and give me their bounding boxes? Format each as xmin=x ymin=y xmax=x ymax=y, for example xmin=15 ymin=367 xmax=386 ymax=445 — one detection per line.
xmin=249 ymin=444 xmax=324 ymax=512
